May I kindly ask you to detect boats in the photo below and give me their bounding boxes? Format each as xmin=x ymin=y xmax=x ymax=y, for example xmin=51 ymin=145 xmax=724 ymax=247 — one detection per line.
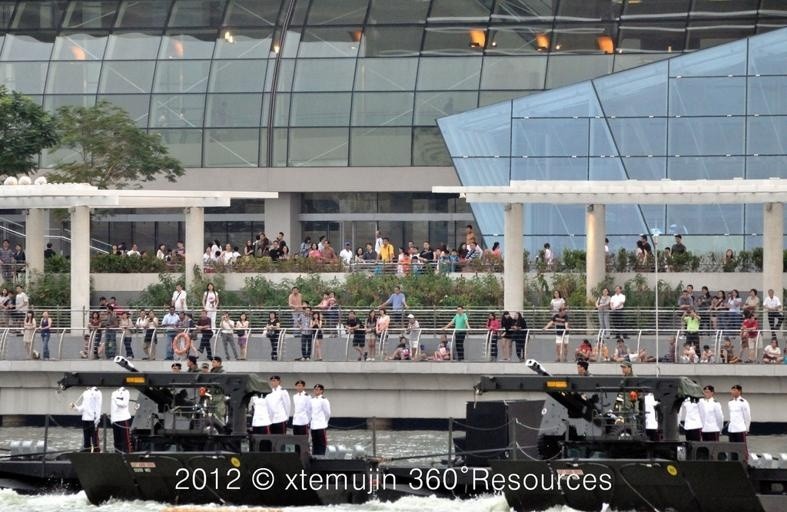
xmin=378 ymin=359 xmax=786 ymax=512
xmin=0 ymin=356 xmax=378 ymax=506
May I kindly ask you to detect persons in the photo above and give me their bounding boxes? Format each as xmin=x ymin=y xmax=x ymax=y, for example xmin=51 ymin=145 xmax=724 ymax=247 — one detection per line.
xmin=0 ymin=223 xmax=787 ymax=466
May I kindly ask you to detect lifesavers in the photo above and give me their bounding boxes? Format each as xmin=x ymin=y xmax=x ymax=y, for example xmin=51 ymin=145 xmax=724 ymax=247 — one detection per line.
xmin=171 ymin=332 xmax=190 ymax=355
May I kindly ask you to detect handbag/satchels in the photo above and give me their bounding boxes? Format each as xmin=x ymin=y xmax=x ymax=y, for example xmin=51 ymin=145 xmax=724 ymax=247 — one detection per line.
xmin=266 ymin=332 xmax=275 ymax=338
xmin=238 ymin=330 xmax=244 ymax=336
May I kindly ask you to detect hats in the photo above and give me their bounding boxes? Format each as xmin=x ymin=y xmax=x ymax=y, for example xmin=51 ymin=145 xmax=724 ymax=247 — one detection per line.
xmin=314 ymin=384 xmax=324 ymax=390
xmin=270 ymin=376 xmax=280 ymax=379
xmin=408 ymin=314 xmax=414 ymax=318
xmin=703 ymin=386 xmax=714 ymax=392
xmin=295 ymin=381 xmax=305 ymax=386
xmin=731 ymin=385 xmax=742 ymax=392
xmin=620 ymin=361 xmax=632 ymax=367
